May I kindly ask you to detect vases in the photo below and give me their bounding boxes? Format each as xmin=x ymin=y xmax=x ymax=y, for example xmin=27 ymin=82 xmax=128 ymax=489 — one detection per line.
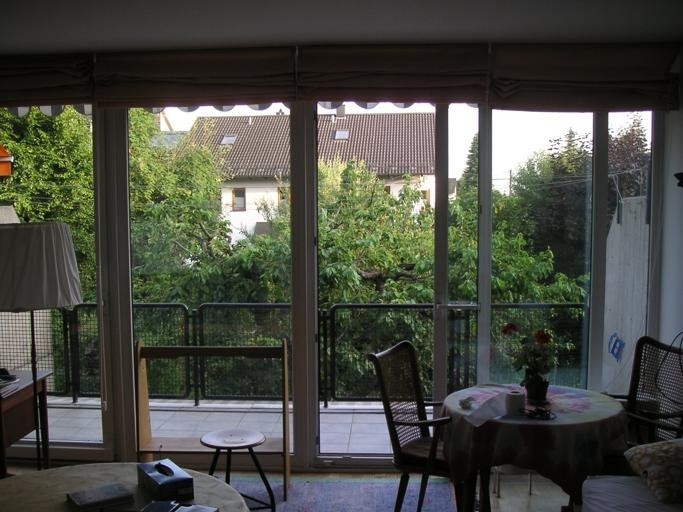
xmin=523 ymin=373 xmax=550 ymax=404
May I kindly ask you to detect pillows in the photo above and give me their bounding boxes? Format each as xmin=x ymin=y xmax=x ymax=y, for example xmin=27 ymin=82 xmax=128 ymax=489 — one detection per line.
xmin=624 ymin=442 xmax=683 ymax=502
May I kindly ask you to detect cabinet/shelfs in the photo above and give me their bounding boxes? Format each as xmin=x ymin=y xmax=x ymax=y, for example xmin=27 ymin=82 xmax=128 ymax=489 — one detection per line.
xmin=132 ymin=338 xmax=291 ymax=500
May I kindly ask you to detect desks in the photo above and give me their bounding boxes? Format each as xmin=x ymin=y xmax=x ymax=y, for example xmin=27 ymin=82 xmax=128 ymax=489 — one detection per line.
xmin=441 ymin=382 xmax=624 ymax=512
xmin=1 ymin=368 xmax=56 ymax=472
xmin=1 ymin=462 xmax=248 ymax=512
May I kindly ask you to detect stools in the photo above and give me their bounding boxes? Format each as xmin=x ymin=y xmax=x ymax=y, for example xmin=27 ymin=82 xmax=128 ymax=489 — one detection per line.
xmin=201 ymin=427 xmax=275 ymax=512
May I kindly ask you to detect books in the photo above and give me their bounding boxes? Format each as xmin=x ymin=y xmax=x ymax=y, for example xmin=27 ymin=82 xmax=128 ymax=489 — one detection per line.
xmin=0 ymin=374 xmax=20 ymax=399
xmin=66 ymin=482 xmax=135 ymax=512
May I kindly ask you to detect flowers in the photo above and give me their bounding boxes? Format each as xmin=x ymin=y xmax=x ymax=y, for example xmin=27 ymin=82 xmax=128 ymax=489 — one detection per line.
xmin=499 ymin=325 xmax=575 ymax=373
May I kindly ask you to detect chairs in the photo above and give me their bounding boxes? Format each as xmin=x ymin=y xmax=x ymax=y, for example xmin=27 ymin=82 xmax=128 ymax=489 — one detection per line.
xmin=367 ymin=339 xmax=455 ymax=512
xmin=601 ymin=335 xmax=683 ymax=474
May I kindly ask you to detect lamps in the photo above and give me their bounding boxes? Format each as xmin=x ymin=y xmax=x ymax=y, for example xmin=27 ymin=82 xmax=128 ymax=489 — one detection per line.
xmin=0 ymin=220 xmax=83 ymax=471
